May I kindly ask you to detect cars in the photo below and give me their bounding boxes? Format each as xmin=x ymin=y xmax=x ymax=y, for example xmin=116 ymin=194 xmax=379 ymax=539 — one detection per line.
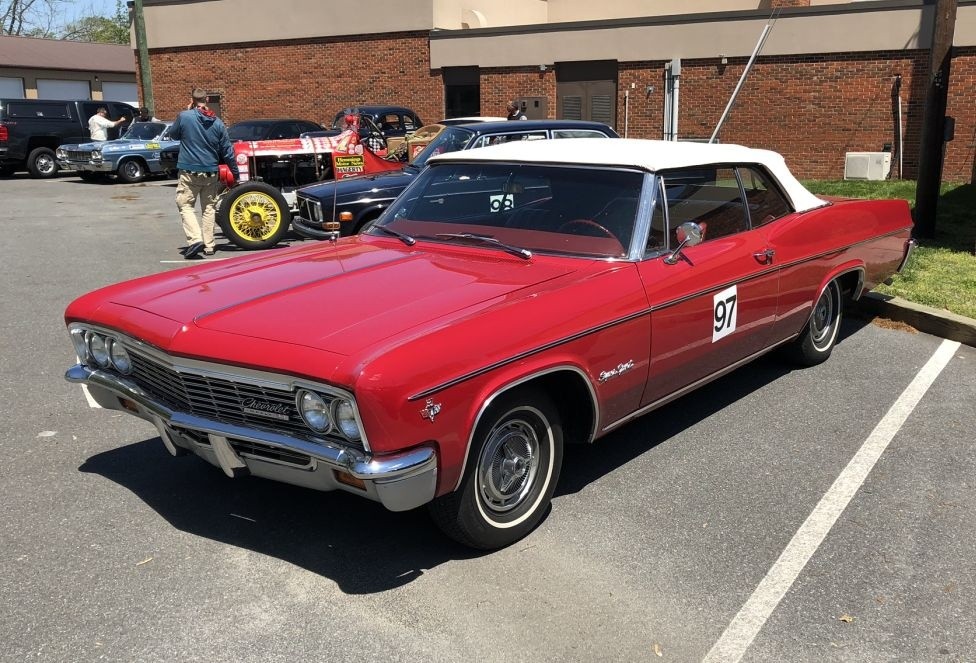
xmin=214 ymin=102 xmax=531 ymax=249
xmin=286 ymin=116 xmax=723 ymax=246
xmin=213 ymin=119 xmax=326 ymax=187
xmin=62 ymin=138 xmax=915 ymax=556
xmin=53 ymin=120 xmax=181 ymax=184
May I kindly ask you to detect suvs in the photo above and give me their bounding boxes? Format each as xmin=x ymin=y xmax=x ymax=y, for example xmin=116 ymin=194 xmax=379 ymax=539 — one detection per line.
xmin=0 ymin=97 xmax=152 ymax=180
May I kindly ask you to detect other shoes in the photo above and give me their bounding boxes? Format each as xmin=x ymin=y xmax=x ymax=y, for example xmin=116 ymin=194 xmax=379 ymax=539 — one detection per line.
xmin=206 ymin=251 xmax=214 ymax=255
xmin=185 ymin=242 xmax=205 ymax=259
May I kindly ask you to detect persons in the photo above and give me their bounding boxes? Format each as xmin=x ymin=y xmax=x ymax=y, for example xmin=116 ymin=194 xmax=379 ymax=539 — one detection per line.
xmin=379 ymin=116 xmax=395 ymax=130
xmin=127 ymin=106 xmax=161 ymax=130
xmin=87 ymin=107 xmax=126 ymax=142
xmin=507 ymin=98 xmax=531 ymax=144
xmin=166 ymin=89 xmax=241 ymax=259
